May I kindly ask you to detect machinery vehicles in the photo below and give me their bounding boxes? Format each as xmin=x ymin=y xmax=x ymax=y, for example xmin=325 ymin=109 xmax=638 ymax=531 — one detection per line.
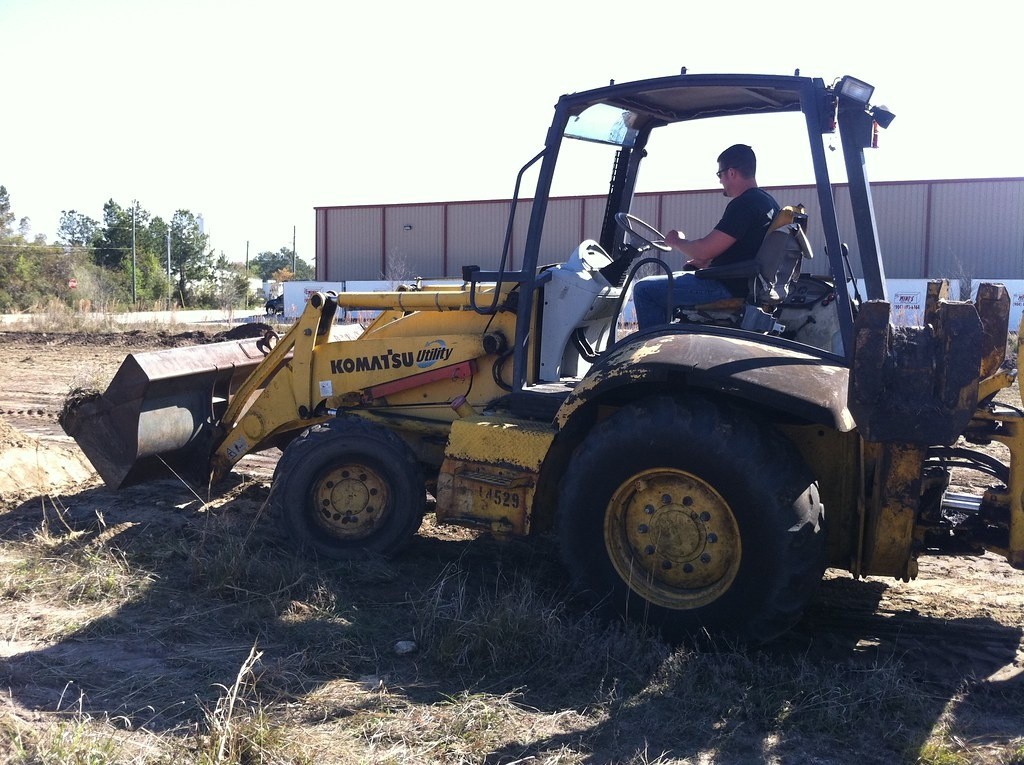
xmin=60 ymin=70 xmax=1024 ymax=637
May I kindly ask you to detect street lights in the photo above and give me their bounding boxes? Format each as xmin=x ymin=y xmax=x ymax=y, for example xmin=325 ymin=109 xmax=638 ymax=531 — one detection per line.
xmin=166 ymin=227 xmax=172 ymax=306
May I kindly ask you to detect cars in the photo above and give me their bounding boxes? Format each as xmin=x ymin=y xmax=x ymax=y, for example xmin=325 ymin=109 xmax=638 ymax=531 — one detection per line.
xmin=265 ymin=295 xmax=284 ymax=316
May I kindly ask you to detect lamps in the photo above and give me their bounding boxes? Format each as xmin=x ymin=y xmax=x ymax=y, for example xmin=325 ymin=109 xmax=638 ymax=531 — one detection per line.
xmin=835 ymin=76 xmax=874 ymax=106
xmin=869 ymin=104 xmax=898 ymax=129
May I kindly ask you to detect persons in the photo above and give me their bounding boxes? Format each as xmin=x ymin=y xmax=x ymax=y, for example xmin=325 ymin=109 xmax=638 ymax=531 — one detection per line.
xmin=633 ymin=144 xmax=782 ymax=330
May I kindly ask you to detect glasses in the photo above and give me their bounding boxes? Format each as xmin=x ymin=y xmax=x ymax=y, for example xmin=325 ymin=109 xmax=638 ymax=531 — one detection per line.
xmin=716 ymin=166 xmax=739 ymax=179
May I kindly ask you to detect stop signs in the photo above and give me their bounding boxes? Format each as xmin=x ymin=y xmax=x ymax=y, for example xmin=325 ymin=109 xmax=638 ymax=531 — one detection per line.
xmin=68 ymin=279 xmax=78 ymax=290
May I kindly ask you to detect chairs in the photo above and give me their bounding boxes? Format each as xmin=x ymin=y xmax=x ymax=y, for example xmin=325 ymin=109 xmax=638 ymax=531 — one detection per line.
xmin=677 ymin=204 xmax=808 ymax=313
